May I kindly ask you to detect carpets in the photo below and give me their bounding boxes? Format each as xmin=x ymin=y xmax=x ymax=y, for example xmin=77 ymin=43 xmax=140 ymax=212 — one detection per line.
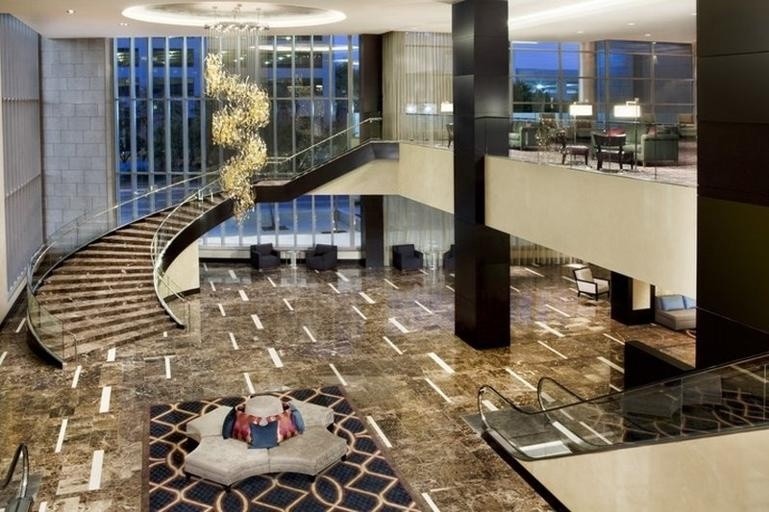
xmin=141 ymin=381 xmax=432 ymax=512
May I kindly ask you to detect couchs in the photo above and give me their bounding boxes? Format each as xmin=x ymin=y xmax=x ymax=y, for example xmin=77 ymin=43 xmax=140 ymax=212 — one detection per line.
xmin=251 ymin=242 xmax=281 ymax=273
xmin=392 ymin=244 xmax=424 ymax=273
xmin=655 ymin=293 xmax=697 ymax=332
xmin=182 ymin=394 xmax=349 ymax=496
xmin=443 ymin=112 xmax=696 ymax=174
xmin=441 ymin=244 xmax=456 ymax=274
xmin=304 ymin=244 xmax=338 ymax=272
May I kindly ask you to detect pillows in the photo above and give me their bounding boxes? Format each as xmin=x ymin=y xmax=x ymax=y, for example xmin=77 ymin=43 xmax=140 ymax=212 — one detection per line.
xmin=683 ymin=296 xmax=696 ymax=309
xmin=220 ymin=397 xmax=306 ymax=450
xmin=661 ymin=296 xmax=685 ymax=311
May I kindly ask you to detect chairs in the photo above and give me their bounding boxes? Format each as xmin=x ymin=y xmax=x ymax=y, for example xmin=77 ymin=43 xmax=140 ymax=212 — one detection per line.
xmin=574 ymin=266 xmax=610 ymax=301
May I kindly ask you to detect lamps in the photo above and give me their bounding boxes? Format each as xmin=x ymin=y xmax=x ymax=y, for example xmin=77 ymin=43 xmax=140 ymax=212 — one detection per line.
xmin=202 ymin=4 xmax=274 ymax=227
xmin=568 ymin=101 xmax=594 ymax=165
xmin=613 ymin=96 xmax=641 ymax=172
